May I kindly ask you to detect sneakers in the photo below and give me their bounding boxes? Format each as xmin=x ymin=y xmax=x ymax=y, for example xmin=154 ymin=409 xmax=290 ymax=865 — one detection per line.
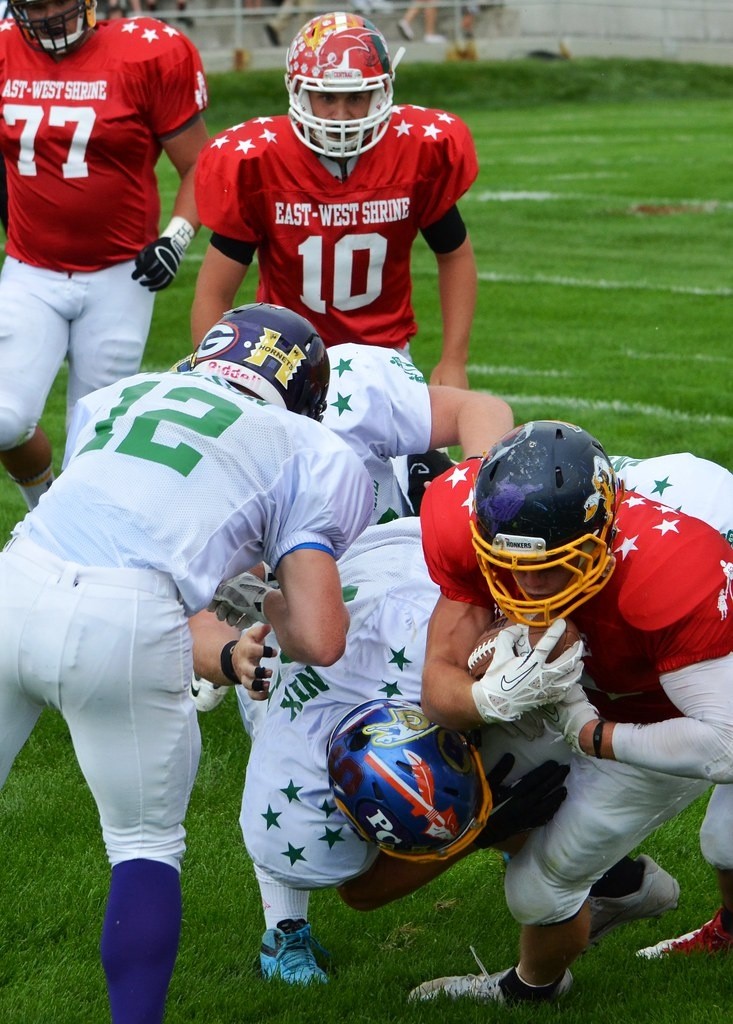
xmin=188 ymin=666 xmax=228 ymax=712
xmin=260 ymin=918 xmax=332 ymax=989
xmin=408 ymin=945 xmax=573 ymax=1010
xmin=635 ymin=903 xmax=733 ymax=961
xmin=579 ymin=854 xmax=680 ymax=953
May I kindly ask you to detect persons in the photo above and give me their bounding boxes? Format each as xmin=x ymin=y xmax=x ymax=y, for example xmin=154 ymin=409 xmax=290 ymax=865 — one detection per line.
xmin=1 ymin=0 xmax=480 ymax=511
xmin=0 ymin=302 xmax=733 ymax=1024
xmin=397 ymin=0 xmax=446 ymax=43
xmin=263 ymin=0 xmax=309 ymax=46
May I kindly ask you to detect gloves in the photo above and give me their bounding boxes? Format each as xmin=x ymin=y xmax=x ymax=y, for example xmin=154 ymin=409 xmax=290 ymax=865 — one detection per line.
xmin=471 ymin=619 xmax=585 ymax=724
xmin=130 ymin=236 xmax=181 ymax=292
xmin=537 ymin=685 xmax=599 ymax=759
xmin=205 ymin=571 xmax=274 ymax=630
xmin=471 ymin=751 xmax=571 ymax=849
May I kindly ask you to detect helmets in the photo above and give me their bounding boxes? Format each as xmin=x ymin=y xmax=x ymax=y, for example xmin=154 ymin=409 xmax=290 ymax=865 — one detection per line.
xmin=324 ymin=698 xmax=492 ymax=864
xmin=284 ymin=12 xmax=394 ymax=159
xmin=474 ymin=419 xmax=617 ymax=627
xmin=9 ymin=0 xmax=96 ymax=55
xmin=189 ymin=302 xmax=330 ymax=417
